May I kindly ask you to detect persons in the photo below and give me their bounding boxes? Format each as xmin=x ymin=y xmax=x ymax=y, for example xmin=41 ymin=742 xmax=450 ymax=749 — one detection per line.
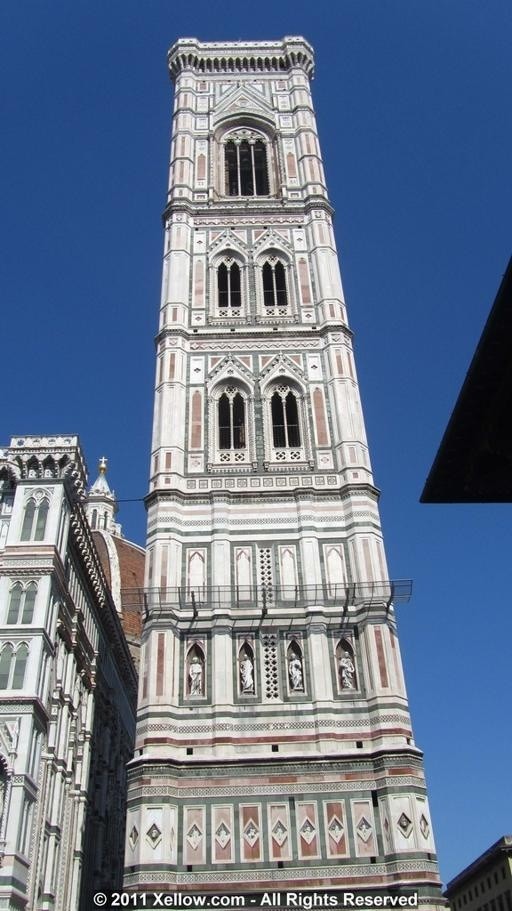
xmin=239 ymin=654 xmax=254 ymax=692
xmin=339 ymin=651 xmax=355 ymax=688
xmin=189 ymin=657 xmax=202 ymax=695
xmin=288 ymin=652 xmax=303 ymax=688
